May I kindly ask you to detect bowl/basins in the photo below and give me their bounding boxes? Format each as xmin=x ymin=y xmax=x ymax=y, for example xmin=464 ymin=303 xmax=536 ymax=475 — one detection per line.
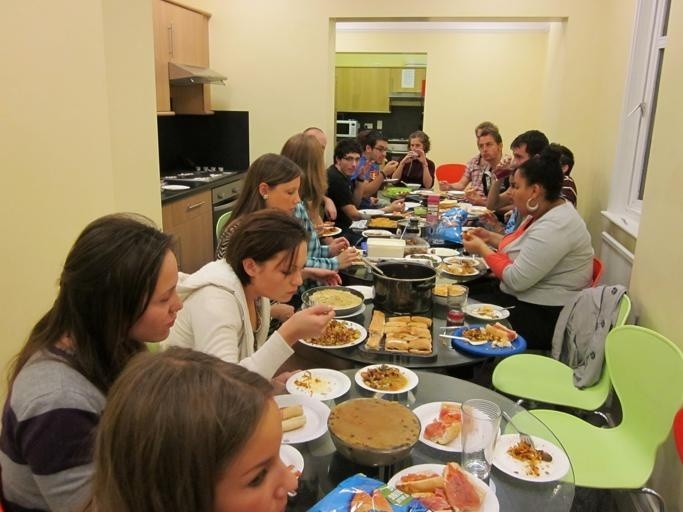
xmin=327 ymin=398 xmax=422 ymax=466
xmin=431 ymin=283 xmax=468 ymax=308
xmin=407 ymin=184 xmax=421 ymax=191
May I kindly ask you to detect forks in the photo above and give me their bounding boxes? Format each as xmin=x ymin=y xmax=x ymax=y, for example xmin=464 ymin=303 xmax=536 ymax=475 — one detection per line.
xmin=438 ymin=334 xmax=488 ymax=345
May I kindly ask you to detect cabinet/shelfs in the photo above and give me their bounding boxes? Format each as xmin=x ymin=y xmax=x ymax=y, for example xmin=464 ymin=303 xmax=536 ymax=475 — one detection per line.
xmin=152 ymin=0 xmax=212 ymax=116
xmin=161 ymin=188 xmax=214 ymax=275
xmin=334 ymin=67 xmax=426 ymax=114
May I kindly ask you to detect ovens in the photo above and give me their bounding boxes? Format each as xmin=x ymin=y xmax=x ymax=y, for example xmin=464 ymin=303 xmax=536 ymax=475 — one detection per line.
xmin=212 ymin=179 xmax=241 ymax=250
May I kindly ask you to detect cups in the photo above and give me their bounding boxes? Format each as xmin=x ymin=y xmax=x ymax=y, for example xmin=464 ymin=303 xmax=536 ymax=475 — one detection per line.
xmin=492 ymin=160 xmax=517 ymax=183
xmin=460 ymin=398 xmax=502 ymax=483
xmin=446 ymin=283 xmax=469 ymax=324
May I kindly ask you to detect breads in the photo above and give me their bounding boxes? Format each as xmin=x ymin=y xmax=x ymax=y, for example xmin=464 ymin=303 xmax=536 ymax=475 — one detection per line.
xmin=438 ymin=421 xmax=462 ymax=445
xmin=366 ymin=310 xmax=432 ymax=355
xmin=279 ymin=405 xmax=305 ymax=432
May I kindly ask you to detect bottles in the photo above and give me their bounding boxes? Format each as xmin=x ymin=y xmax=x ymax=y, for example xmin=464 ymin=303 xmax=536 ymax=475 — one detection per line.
xmin=401 ymin=218 xmax=420 ymax=239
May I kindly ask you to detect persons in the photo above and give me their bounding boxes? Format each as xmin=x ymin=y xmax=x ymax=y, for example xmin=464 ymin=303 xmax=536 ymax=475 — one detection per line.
xmin=159 ymin=208 xmax=336 ymax=396
xmin=0 ymin=213 xmax=184 ymax=512
xmin=439 ymin=121 xmax=595 ymax=351
xmin=92 ymin=346 xmax=298 ymax=512
xmin=391 ymin=130 xmax=435 ymax=189
xmin=216 ymin=127 xmax=405 ymax=334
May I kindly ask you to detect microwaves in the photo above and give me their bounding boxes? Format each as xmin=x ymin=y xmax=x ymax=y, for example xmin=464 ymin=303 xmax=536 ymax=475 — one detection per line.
xmin=336 ymin=119 xmax=360 ymax=139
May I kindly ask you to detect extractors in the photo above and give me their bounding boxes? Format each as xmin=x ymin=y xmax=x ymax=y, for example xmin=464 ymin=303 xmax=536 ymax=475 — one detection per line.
xmin=168 ymin=61 xmax=228 ymax=87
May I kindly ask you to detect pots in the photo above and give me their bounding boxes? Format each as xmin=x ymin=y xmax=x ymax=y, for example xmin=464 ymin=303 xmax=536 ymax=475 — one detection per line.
xmin=370 ymin=261 xmax=437 ymax=314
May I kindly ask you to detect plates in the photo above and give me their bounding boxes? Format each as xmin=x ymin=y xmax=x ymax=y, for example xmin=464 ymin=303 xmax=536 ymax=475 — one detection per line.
xmin=287 ymin=368 xmax=352 ymax=401
xmin=383 ymin=179 xmax=399 ymax=182
xmin=272 ymin=393 xmax=331 ymax=445
xmin=447 ymin=190 xmax=465 ymax=197
xmin=299 ymin=284 xmax=373 ymax=349
xmin=357 ymin=208 xmax=385 ymax=216
xmin=354 ymin=365 xmax=420 ymax=395
xmin=405 ymin=202 xmax=420 ymax=209
xmin=279 ymin=445 xmax=306 ymax=478
xmin=390 ymin=463 xmax=501 ymax=512
xmin=361 ymin=229 xmax=392 ymax=238
xmin=318 ymin=227 xmax=343 ymax=238
xmin=452 ymin=302 xmax=528 ymax=356
xmin=486 ymin=432 xmax=571 ymax=482
xmin=412 ymin=401 xmax=501 ymax=454
xmin=161 ymin=185 xmax=191 ymax=191
xmin=405 ymin=247 xmax=480 ymax=276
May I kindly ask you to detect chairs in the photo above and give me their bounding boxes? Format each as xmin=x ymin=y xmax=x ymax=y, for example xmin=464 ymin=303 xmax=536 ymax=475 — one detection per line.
xmin=592 ymin=258 xmax=603 ymax=287
xmin=436 ymin=163 xmax=469 ymax=188
xmin=502 ymin=324 xmax=682 ymax=512
xmin=492 ymin=290 xmax=631 ymax=426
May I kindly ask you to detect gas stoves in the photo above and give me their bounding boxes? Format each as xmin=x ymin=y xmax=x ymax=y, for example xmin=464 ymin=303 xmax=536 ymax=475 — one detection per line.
xmin=163 ymin=167 xmax=240 ymax=183
